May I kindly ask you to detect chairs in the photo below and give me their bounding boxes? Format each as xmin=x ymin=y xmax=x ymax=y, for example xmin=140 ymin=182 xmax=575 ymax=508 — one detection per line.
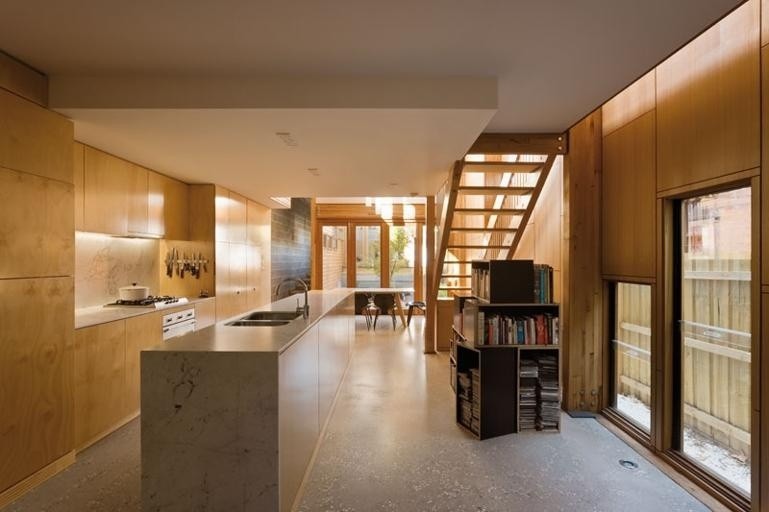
xmin=354 ymin=293 xmax=427 ymax=331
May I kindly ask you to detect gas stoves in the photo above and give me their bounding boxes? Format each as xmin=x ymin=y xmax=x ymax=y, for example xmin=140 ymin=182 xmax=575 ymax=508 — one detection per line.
xmin=103 ymin=294 xmax=189 ymax=309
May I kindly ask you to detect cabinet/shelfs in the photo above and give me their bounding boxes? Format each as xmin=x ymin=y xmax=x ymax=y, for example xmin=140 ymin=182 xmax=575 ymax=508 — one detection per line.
xmin=72 ymin=140 xmax=189 ymax=240
xmin=195 ymin=298 xmax=216 ymax=332
xmin=0 ymin=86 xmax=76 ymax=506
xmin=448 ymin=257 xmax=563 ymax=442
xmin=72 ymin=311 xmax=163 ymax=456
xmin=190 ymin=183 xmax=272 ymax=322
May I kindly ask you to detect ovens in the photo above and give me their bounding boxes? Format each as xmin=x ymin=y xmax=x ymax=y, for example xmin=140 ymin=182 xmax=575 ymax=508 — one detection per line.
xmin=162 ymin=307 xmax=196 ymax=340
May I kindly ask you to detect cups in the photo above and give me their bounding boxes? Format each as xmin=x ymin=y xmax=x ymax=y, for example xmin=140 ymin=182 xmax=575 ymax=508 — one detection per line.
xmin=200 ymin=289 xmax=209 ymax=298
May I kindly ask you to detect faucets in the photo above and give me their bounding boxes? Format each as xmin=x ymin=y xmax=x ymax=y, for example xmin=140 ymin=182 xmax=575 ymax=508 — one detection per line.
xmin=275 ymin=276 xmax=309 ymax=318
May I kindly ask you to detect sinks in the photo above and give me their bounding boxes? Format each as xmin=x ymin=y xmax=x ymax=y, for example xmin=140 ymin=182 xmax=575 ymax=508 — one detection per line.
xmin=228 ymin=319 xmax=291 ymax=328
xmin=237 ymin=309 xmax=305 ymax=320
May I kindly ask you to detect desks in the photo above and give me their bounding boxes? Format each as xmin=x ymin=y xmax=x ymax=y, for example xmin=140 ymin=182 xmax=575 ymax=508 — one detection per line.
xmin=330 ymin=287 xmax=415 ymax=328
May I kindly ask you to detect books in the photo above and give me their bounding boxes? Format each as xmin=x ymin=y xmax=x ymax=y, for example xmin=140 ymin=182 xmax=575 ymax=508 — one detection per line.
xmin=477 ymin=312 xmax=559 ymax=345
xmin=459 ymin=369 xmax=479 ymax=436
xmin=471 ymin=268 xmax=490 ymax=300
xmin=520 ymin=355 xmax=559 ymax=431
xmin=534 ymin=264 xmax=554 ymax=304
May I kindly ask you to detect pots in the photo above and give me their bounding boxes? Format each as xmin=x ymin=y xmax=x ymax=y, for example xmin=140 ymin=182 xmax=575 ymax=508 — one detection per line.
xmin=118 ymin=283 xmax=150 ymax=302
